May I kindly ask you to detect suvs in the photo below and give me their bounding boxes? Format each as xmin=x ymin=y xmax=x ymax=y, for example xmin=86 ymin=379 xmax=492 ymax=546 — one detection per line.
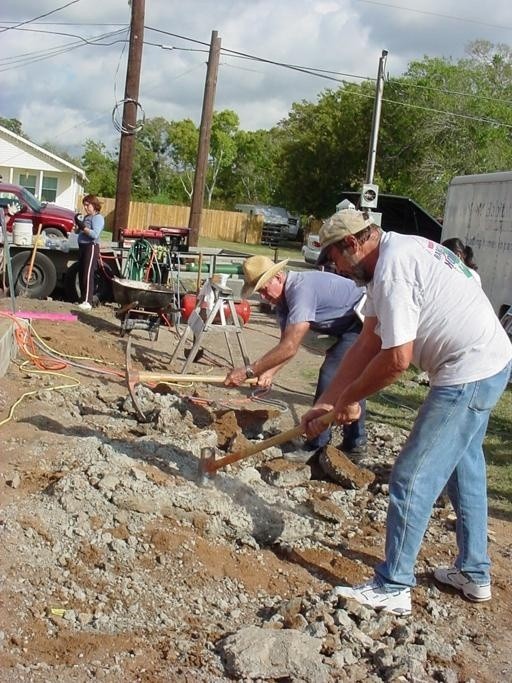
xmin=1 ymin=183 xmax=78 ymax=238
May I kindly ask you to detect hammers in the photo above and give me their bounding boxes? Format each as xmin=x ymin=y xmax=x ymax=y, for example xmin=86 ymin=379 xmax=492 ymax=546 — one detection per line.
xmin=200 ymin=402 xmax=359 ymax=480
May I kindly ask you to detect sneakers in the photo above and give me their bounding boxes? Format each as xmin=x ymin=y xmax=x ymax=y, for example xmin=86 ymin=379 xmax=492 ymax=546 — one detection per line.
xmin=434 ymin=569 xmax=492 ymax=602
xmin=332 ymin=576 xmax=411 ymax=616
xmin=77 ymin=301 xmax=92 ymax=309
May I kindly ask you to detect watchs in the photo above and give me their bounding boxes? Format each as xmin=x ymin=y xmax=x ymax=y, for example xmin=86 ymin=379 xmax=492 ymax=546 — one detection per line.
xmin=245 ymin=365 xmax=255 ymax=378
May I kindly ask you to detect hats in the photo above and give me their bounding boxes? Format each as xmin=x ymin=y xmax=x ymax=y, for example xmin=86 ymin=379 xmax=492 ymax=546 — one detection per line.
xmin=314 ymin=208 xmax=374 ymax=265
xmin=241 ymin=255 xmax=289 ymax=299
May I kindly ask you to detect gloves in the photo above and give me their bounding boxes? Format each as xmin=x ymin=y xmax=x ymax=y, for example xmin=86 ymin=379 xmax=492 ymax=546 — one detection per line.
xmin=7 ymin=201 xmax=21 ymax=215
xmin=75 ymin=213 xmax=86 ymax=231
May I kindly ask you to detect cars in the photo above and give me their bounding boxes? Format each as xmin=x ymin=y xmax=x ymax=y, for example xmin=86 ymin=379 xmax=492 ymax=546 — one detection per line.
xmin=303 ymin=235 xmax=329 ymax=263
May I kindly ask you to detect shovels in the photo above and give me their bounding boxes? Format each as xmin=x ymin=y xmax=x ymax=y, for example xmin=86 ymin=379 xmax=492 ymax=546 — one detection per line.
xmin=19 ymin=224 xmax=42 ymax=299
xmin=184 ymin=251 xmax=203 ymax=363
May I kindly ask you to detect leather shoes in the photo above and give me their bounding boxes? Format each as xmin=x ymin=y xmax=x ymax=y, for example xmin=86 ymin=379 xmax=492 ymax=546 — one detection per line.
xmin=282 ymin=433 xmax=368 ymax=463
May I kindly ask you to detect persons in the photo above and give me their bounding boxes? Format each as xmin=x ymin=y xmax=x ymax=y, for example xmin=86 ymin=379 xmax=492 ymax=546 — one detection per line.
xmin=300 ymin=208 xmax=512 ymax=616
xmin=73 ymin=194 xmax=105 ymax=311
xmin=441 ymin=237 xmax=482 ymax=287
xmin=223 ymin=254 xmax=369 ymax=463
xmin=0 ymin=201 xmax=21 ymax=225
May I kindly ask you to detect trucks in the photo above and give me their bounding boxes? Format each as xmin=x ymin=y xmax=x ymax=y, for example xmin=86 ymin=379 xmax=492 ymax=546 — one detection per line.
xmin=441 ymin=171 xmax=511 ymax=335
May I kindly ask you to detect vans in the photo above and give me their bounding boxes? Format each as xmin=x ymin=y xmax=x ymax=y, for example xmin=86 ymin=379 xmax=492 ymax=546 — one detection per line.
xmin=234 ymin=202 xmax=303 ymax=245
xmin=338 ymin=190 xmax=442 ymax=244
